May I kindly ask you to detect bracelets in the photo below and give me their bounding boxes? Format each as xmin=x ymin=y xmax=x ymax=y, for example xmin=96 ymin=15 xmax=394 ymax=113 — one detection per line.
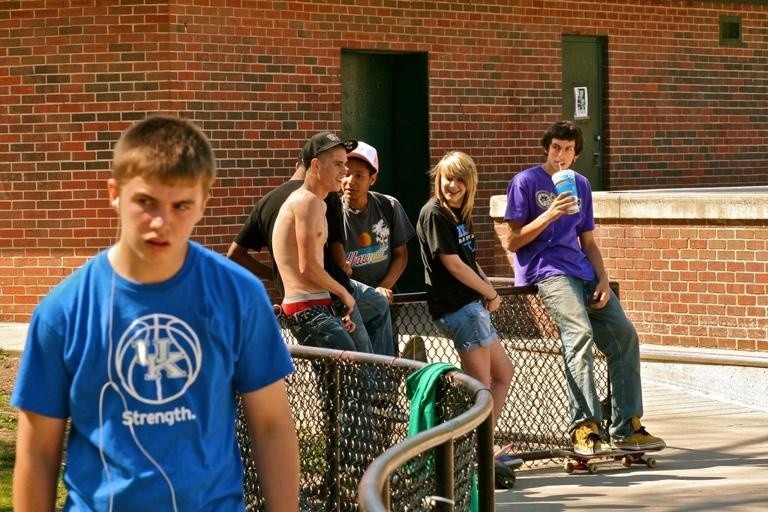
xmin=486 ymin=291 xmax=500 ymax=302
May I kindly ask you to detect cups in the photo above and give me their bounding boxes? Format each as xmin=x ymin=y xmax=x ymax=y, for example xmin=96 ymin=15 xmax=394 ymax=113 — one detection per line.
xmin=552 ymin=169 xmax=582 ymax=216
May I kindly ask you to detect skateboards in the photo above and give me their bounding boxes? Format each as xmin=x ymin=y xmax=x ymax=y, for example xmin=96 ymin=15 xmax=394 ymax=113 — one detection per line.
xmin=550 ymin=447 xmax=667 ymax=474
xmin=401 ymin=336 xmax=427 ymax=381
xmin=494 ymin=443 xmax=516 ymax=488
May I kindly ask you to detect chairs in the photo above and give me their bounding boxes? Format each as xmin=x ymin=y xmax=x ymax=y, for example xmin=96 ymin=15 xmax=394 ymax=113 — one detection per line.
xmin=230 ymin=346 xmax=496 ymax=512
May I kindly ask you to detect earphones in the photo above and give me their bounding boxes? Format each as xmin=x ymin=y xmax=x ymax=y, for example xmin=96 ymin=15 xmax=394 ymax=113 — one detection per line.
xmin=112 ymin=197 xmax=120 ymax=207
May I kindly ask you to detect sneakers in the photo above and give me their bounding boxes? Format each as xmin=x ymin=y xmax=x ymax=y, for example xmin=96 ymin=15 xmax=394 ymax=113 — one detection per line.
xmin=609 ymin=416 xmax=667 ymax=450
xmin=572 ymin=420 xmax=612 ymax=456
xmin=493 ymin=455 xmax=523 ymax=469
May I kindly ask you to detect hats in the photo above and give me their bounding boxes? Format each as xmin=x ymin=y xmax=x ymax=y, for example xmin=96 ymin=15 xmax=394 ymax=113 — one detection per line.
xmin=303 ymin=131 xmax=358 ymax=170
xmin=346 ymin=141 xmax=379 ymax=176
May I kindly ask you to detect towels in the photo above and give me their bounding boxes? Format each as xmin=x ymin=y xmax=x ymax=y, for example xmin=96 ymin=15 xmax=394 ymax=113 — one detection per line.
xmin=404 ymin=360 xmax=481 ymax=512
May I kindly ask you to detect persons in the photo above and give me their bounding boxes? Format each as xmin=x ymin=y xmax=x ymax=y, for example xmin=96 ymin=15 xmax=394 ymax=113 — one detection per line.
xmin=501 ymin=119 xmax=668 ymax=457
xmin=339 ymin=140 xmax=416 ymax=449
xmin=416 ymin=148 xmax=525 ymax=470
xmin=10 ymin=113 xmax=303 ymax=512
xmin=225 ymin=148 xmax=409 ymax=423
xmin=270 ymin=132 xmax=376 ymax=478
